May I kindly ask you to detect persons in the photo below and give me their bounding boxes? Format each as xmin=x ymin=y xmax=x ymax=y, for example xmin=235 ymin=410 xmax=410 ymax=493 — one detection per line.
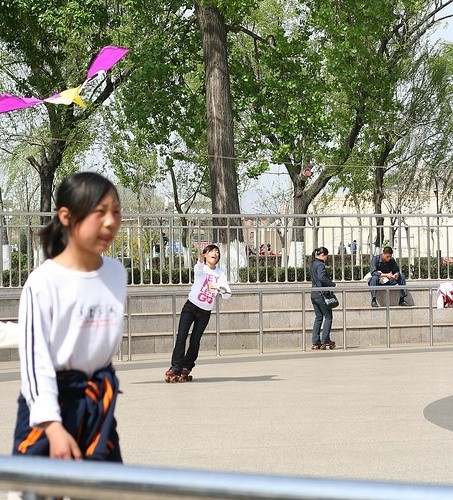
xmin=260 ymin=244 xmax=266 ymax=255
xmin=165 ymin=245 xmax=232 ymax=378
xmin=348 ymin=240 xmax=357 ymax=254
xmin=267 ymin=244 xmax=272 ymax=255
xmin=368 ymin=247 xmax=412 ymax=307
xmin=162 ymin=233 xmax=169 ymax=258
xmin=338 ymin=242 xmax=346 ymax=254
xmin=311 ymin=247 xmax=336 ymax=346
xmin=12 ymin=172 xmax=128 ymax=499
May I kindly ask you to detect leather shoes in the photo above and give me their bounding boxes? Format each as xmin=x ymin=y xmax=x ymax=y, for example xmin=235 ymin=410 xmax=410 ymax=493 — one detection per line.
xmin=398 ymin=300 xmax=411 ymax=306
xmin=371 ymin=301 xmax=378 ymax=307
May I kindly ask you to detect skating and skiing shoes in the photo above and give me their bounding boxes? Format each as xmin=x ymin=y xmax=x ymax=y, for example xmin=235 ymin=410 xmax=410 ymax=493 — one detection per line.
xmin=165 ymin=368 xmax=180 ymax=382
xmin=311 ymin=341 xmax=322 ymax=350
xmin=175 ymin=368 xmax=192 ymax=382
xmin=321 ymin=339 xmax=335 ymax=350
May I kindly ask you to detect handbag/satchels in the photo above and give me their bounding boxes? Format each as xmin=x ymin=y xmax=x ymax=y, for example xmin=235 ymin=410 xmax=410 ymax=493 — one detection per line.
xmin=323 ymin=295 xmax=338 ymax=308
